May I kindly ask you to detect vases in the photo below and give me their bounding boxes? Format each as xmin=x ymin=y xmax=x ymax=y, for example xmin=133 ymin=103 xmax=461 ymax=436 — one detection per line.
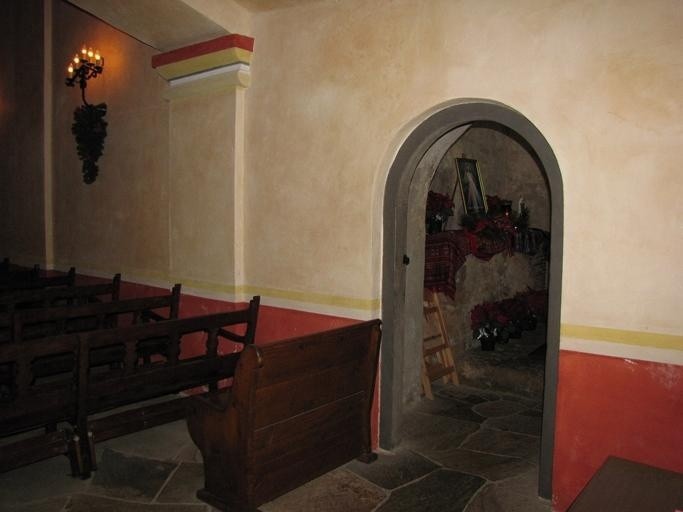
xmin=427 ymin=222 xmax=446 ymax=234
xmin=510 ymin=325 xmax=524 ymax=339
xmin=498 ymin=328 xmax=514 ymax=343
xmin=480 ymin=334 xmax=498 ymax=350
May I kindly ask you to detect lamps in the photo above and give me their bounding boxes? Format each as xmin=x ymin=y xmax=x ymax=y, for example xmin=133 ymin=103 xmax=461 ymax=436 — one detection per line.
xmin=64 ymin=46 xmax=108 ymax=184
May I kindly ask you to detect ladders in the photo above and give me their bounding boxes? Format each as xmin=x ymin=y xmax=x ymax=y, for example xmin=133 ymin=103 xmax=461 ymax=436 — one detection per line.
xmin=420 ymin=290 xmax=461 ymax=400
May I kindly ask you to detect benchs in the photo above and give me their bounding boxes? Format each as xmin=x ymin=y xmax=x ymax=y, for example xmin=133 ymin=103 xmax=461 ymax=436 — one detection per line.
xmin=0 ymin=260 xmax=382 ymax=512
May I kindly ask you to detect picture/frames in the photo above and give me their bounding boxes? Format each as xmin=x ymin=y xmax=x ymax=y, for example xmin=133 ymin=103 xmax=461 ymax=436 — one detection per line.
xmin=455 ymin=156 xmax=489 ymax=217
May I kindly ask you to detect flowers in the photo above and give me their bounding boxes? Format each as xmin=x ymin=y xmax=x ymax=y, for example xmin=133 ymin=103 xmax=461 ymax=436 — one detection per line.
xmin=424 ymin=192 xmax=454 ymax=221
xmin=468 ymin=288 xmax=545 ymax=334
